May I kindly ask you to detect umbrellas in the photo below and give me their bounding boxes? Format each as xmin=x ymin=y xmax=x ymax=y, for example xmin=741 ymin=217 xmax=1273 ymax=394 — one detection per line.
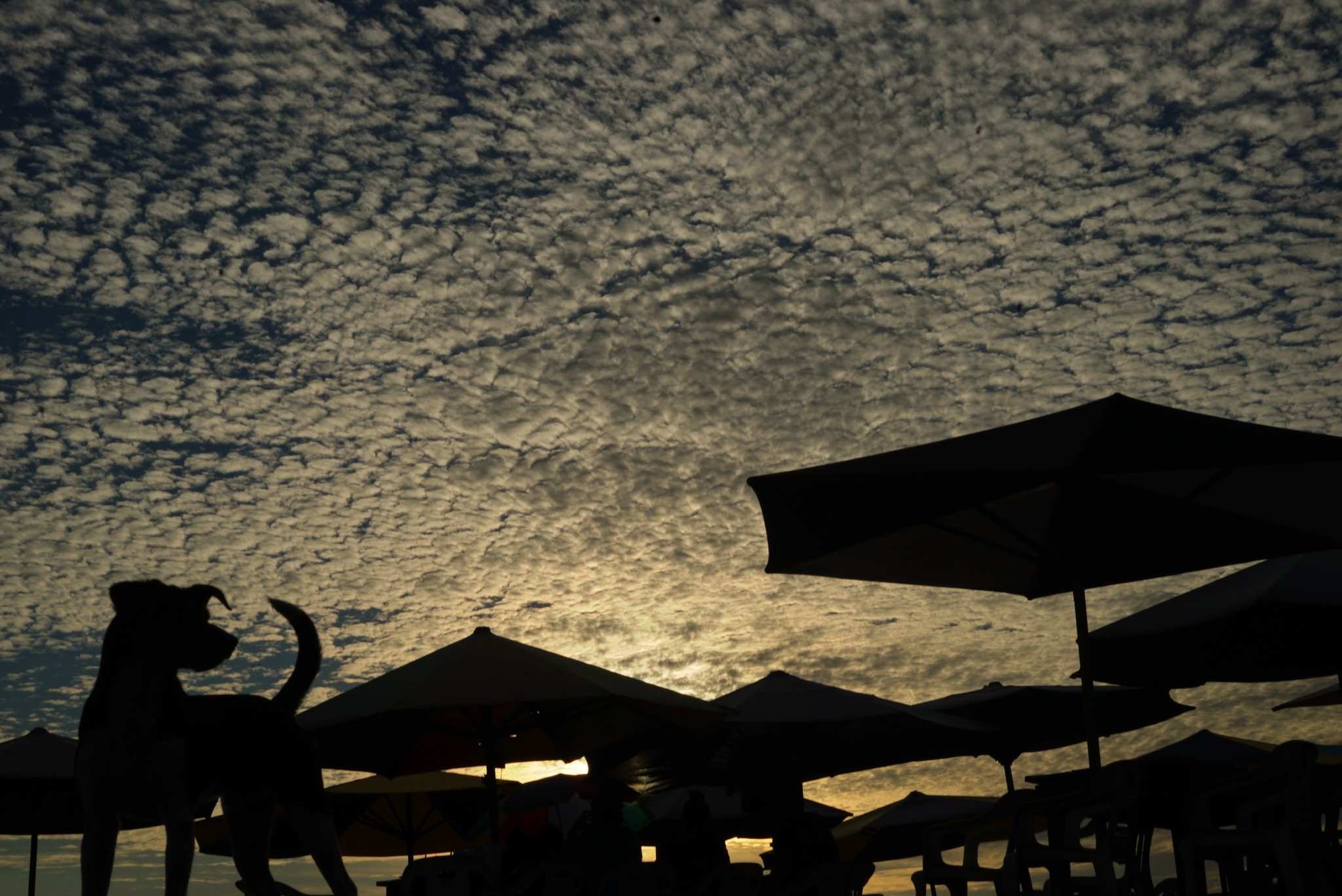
xmin=1082 ymin=549 xmax=1340 ymax=690
xmin=747 ymin=392 xmax=1342 ymax=896
xmin=475 ymin=670 xmax=1342 ymax=896
xmin=0 ymin=726 xmax=218 ymax=896
xmin=326 ymin=767 xmax=521 ymax=864
xmin=298 ymin=625 xmax=707 ymax=849
xmin=195 ymin=793 xmax=372 ymax=859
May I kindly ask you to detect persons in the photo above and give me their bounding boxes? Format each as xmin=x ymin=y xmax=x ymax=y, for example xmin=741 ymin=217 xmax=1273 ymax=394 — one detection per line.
xmin=575 ymin=789 xmax=638 ymax=863
xmin=664 ymin=799 xmax=725 ymax=877
xmin=510 ymin=824 xmax=565 ymax=883
xmin=499 ymin=826 xmax=532 ymax=888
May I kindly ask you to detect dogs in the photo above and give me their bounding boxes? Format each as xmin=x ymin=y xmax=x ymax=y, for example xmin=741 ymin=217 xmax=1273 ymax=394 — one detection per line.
xmin=71 ymin=576 xmax=362 ymax=896
